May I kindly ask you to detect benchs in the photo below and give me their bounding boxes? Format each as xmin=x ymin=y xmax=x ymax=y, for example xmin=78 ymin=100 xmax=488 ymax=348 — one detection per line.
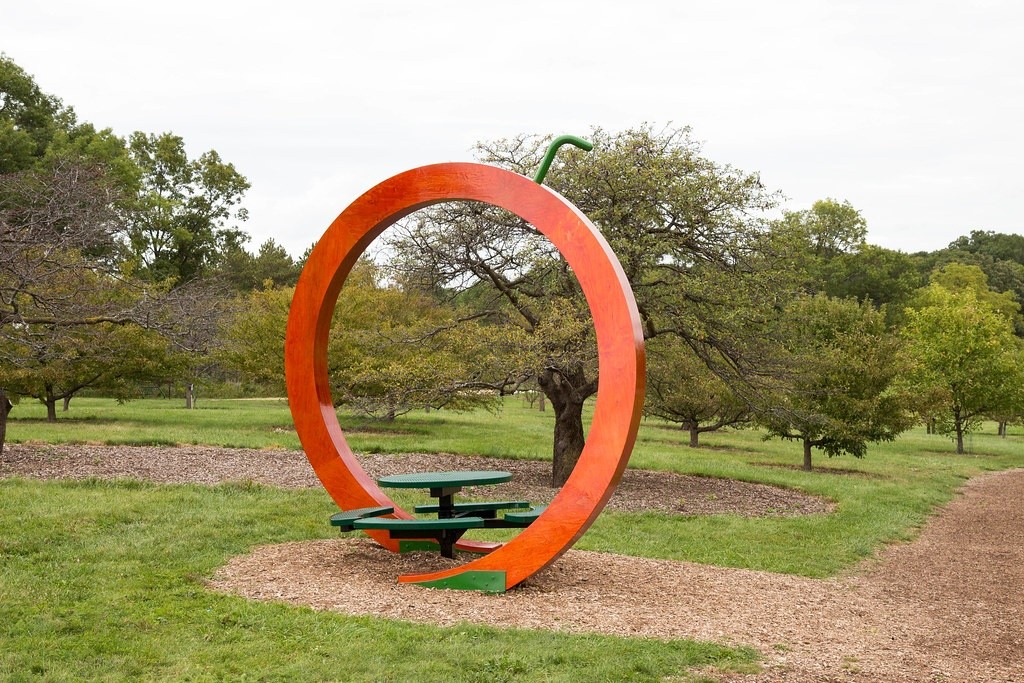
xmin=328 ymin=499 xmax=549 ymax=544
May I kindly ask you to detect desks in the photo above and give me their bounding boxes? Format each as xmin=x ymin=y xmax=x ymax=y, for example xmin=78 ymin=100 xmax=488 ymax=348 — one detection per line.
xmin=376 ymin=469 xmax=514 ymax=558
